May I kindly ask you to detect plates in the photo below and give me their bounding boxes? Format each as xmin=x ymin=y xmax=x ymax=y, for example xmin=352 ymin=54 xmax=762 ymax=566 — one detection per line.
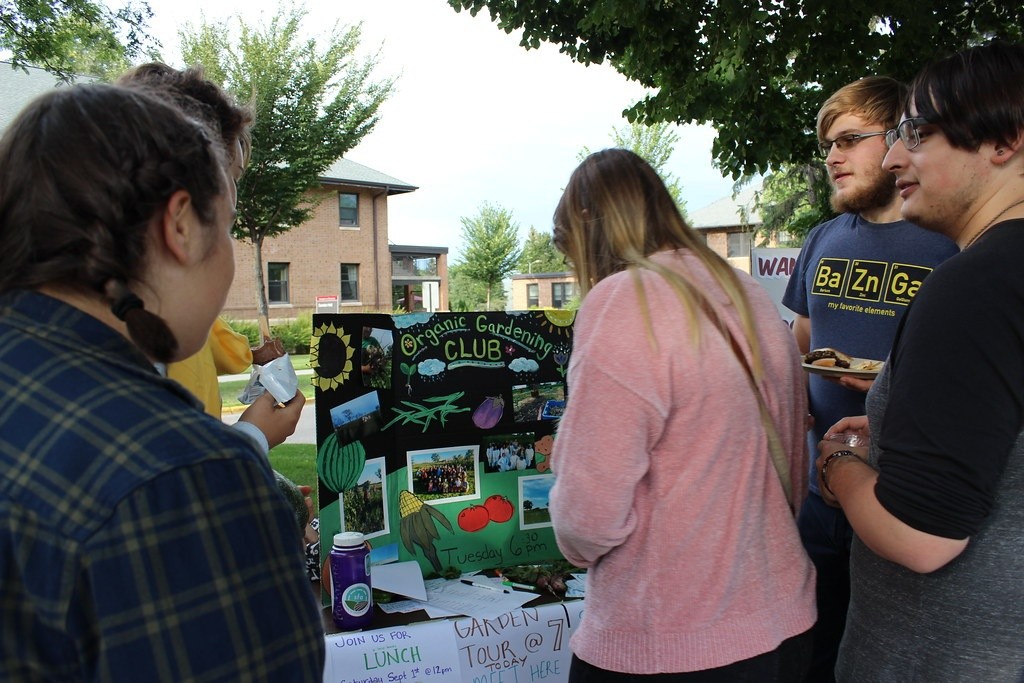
xmin=800 ymin=357 xmax=886 ymax=379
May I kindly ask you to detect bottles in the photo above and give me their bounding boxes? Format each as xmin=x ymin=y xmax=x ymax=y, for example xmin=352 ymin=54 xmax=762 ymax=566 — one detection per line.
xmin=328 ymin=531 xmax=374 ymax=630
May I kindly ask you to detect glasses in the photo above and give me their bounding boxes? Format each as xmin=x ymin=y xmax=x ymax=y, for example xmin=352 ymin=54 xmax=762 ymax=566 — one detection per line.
xmin=820 ymin=131 xmax=886 ymax=157
xmin=885 ymin=118 xmax=935 ymax=150
xmin=562 ymin=253 xmax=575 ymax=269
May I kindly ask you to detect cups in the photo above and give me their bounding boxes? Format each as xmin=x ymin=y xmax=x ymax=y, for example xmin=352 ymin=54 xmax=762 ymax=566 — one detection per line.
xmin=822 ymin=432 xmax=871 ymax=508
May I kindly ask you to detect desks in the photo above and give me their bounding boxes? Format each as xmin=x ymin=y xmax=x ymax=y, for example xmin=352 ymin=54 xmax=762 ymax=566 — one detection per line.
xmin=310 ymin=560 xmax=588 ymax=683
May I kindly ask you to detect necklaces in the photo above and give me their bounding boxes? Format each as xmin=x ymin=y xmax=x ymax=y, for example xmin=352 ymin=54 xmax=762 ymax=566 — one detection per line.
xmin=961 ymin=199 xmax=1024 ymax=252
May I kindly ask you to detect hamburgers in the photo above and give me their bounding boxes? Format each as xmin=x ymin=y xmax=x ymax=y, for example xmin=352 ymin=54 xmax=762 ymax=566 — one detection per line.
xmin=802 ymin=347 xmax=852 ymax=369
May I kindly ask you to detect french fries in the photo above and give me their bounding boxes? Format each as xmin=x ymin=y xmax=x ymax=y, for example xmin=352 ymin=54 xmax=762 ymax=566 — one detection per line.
xmin=855 ymin=360 xmax=882 ymax=370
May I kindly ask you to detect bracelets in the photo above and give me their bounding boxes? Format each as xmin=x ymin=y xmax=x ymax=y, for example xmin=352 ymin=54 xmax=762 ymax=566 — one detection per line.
xmin=821 ymin=450 xmax=864 ymax=497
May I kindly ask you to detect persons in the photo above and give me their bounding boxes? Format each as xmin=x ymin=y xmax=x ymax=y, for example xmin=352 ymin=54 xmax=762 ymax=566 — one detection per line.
xmin=780 ymin=39 xmax=1024 ymax=683
xmin=549 ymin=148 xmax=821 ymax=683
xmin=1 ymin=62 xmax=325 ymax=683
